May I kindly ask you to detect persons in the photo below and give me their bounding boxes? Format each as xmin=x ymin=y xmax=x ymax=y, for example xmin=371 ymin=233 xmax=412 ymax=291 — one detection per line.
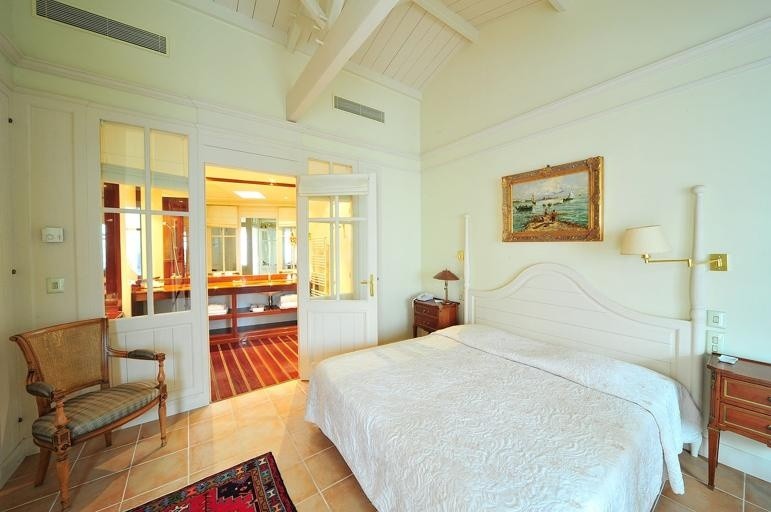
xmin=532 ymin=206 xmax=557 ymax=222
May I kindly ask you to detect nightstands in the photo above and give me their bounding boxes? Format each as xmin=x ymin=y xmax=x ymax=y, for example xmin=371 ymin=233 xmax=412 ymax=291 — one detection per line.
xmin=707 ymin=353 xmax=771 ymax=491
xmin=412 ymin=298 xmax=460 ymax=338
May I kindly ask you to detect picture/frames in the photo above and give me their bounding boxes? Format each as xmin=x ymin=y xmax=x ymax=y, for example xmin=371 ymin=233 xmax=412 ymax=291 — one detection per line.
xmin=503 ymin=157 xmax=603 ymax=242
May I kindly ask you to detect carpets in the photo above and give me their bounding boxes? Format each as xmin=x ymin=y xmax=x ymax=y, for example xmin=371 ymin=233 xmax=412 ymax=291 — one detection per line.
xmin=124 ymin=451 xmax=297 ymax=512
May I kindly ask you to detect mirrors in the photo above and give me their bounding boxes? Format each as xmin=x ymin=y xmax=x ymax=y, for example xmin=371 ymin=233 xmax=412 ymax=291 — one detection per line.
xmin=239 ymin=217 xmax=278 ymax=274
xmin=278 ymin=222 xmax=296 ymax=274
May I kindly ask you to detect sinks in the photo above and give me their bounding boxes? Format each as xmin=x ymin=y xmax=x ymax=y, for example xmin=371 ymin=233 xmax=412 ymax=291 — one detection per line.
xmin=256 ymin=281 xmax=286 ymax=296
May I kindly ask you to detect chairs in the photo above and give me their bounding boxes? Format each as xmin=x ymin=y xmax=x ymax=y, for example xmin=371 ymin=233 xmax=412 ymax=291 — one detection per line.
xmin=9 ymin=316 xmax=168 ymax=511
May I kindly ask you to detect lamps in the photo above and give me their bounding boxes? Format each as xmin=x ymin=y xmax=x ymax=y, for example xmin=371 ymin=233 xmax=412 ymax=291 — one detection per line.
xmin=434 ymin=269 xmax=459 ymax=304
xmin=620 ymin=225 xmax=726 ymax=272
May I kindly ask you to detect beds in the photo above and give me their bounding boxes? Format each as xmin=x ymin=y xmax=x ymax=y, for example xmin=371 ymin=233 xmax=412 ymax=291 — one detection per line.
xmin=303 ymin=324 xmax=704 ymax=512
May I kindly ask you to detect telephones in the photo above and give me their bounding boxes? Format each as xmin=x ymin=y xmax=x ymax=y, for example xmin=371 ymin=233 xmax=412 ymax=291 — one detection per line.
xmin=416 ymin=293 xmax=433 ymax=302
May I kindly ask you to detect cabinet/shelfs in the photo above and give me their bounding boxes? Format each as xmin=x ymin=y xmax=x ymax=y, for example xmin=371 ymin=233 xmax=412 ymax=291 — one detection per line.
xmin=131 ymin=285 xmax=298 ymax=349
xmin=207 ymin=205 xmax=239 ymax=275
